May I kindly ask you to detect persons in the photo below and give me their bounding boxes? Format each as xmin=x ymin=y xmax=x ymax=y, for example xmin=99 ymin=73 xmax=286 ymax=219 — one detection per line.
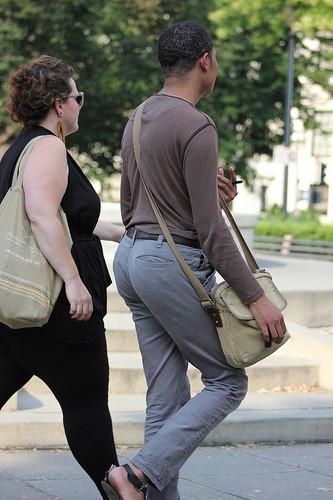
xmin=0 ymin=55 xmax=126 ymax=500
xmin=101 ymin=22 xmax=288 ymax=500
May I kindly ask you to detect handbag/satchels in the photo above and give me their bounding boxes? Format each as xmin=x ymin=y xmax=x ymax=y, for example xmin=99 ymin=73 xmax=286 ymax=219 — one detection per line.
xmin=0 ymin=133 xmax=77 ymax=331
xmin=207 ymin=268 xmax=291 ymax=369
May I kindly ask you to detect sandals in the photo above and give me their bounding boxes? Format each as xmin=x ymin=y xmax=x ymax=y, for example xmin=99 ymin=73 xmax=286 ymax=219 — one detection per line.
xmin=98 ymin=465 xmax=149 ymax=498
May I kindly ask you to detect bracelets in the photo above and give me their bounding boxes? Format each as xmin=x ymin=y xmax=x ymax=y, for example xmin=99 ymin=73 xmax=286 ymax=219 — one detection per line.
xmin=118 ymin=231 xmax=126 ymax=243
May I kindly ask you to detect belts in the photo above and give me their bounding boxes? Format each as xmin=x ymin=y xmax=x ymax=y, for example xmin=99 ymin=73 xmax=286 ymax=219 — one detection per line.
xmin=123 ymin=224 xmax=208 ymax=249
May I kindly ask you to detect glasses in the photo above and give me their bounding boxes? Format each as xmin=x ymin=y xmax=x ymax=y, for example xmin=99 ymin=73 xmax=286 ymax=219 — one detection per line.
xmin=62 ymin=90 xmax=84 ymax=109
